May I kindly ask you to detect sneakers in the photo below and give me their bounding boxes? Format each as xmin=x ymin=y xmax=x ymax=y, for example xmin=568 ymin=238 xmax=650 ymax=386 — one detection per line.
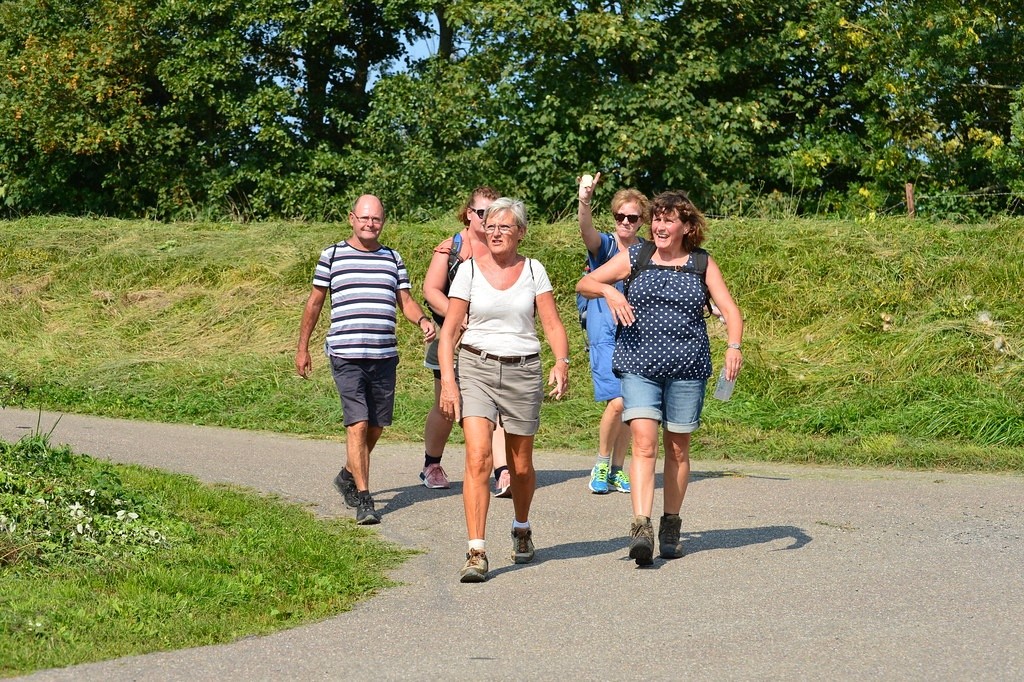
xmin=607 ymin=471 xmax=631 ymax=493
xmin=511 ymin=517 xmax=535 ymax=564
xmin=658 ymin=514 xmax=682 ymax=558
xmin=494 ymin=470 xmax=512 ymax=498
xmin=419 ymin=460 xmax=450 ymax=489
xmin=333 ymin=466 xmax=359 ymax=510
xmin=355 ymin=489 xmax=380 ymax=525
xmin=589 ymin=461 xmax=609 ymax=494
xmin=460 ymin=548 xmax=488 ymax=582
xmin=629 ymin=515 xmax=654 ymax=566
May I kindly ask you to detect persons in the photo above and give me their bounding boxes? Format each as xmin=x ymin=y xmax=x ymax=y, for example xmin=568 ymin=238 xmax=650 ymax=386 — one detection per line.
xmin=423 ymin=187 xmax=510 ymax=497
xmin=575 ymin=192 xmax=742 ymax=566
xmin=296 ymin=194 xmax=436 ymax=524
xmin=578 ymin=172 xmax=650 ymax=493
xmin=437 ymin=197 xmax=569 ymax=582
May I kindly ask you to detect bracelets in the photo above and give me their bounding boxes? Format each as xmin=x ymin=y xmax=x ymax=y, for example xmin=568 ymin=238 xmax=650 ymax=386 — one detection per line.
xmin=579 ymin=198 xmax=591 ymax=205
xmin=418 ymin=316 xmax=431 ymax=327
xmin=728 ymin=344 xmax=741 ymax=350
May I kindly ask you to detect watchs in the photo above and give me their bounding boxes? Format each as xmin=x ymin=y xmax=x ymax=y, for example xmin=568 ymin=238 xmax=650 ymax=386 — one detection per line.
xmin=557 ymin=358 xmax=569 ymax=364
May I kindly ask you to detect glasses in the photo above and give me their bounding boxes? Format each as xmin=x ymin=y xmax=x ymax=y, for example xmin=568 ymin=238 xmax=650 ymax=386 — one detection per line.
xmin=485 ymin=225 xmax=521 ymax=234
xmin=353 ymin=213 xmax=384 ymax=223
xmin=613 ymin=213 xmax=643 ymax=223
xmin=469 ymin=206 xmax=485 ymax=219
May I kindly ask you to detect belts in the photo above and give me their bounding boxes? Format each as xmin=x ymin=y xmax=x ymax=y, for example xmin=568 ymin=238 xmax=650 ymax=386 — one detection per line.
xmin=463 ymin=345 xmax=539 ymax=363
